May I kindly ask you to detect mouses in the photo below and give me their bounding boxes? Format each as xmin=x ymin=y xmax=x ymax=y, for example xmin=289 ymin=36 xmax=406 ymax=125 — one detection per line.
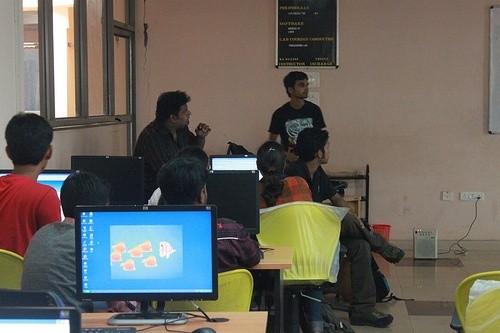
xmin=193 ymin=327 xmax=216 ymax=333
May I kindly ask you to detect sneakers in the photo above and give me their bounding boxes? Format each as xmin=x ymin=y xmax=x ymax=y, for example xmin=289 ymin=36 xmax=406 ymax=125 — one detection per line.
xmin=349 ymin=308 xmax=393 ymax=326
xmin=372 ymin=238 xmax=405 ymax=263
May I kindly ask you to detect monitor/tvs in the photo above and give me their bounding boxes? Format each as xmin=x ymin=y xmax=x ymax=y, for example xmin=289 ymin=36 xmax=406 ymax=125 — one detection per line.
xmin=74 ymin=203 xmax=217 ymax=323
xmin=70 ymin=155 xmax=144 ymax=205
xmin=208 ymin=154 xmax=263 ymax=180
xmin=206 ymin=170 xmax=259 ymax=234
xmin=0 ymin=169 xmax=73 ymax=223
xmin=0 ymin=305 xmax=81 ymax=333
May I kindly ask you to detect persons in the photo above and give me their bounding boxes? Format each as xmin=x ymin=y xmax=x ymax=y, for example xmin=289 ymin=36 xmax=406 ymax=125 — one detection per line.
xmin=141 ymin=146 xmax=212 ymax=208
xmin=0 ymin=111 xmax=63 ymax=260
xmin=268 ymin=70 xmax=330 ymax=170
xmin=157 ymin=158 xmax=264 ymax=311
xmin=134 ymin=90 xmax=212 ymax=204
xmin=256 ymin=127 xmax=406 ymax=333
xmin=22 ymin=171 xmax=141 ymax=315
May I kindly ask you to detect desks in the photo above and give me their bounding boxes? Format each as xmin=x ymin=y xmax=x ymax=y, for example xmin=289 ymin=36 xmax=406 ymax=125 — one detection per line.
xmin=79 ymin=311 xmax=268 ymax=333
xmin=249 ymin=247 xmax=294 ymax=333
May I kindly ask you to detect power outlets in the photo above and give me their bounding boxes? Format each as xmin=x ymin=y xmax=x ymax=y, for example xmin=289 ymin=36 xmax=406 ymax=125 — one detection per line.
xmin=461 ymin=192 xmax=484 ymax=200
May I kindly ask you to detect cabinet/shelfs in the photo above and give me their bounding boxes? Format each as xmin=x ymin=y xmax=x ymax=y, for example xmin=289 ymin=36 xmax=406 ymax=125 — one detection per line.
xmin=327 ymin=164 xmax=369 ymax=232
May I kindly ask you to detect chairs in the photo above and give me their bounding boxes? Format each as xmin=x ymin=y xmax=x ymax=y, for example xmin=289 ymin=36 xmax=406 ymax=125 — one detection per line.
xmin=455 ymin=271 xmax=500 ymax=333
xmin=259 ymin=201 xmax=341 ymax=333
xmin=151 ymin=269 xmax=254 ymax=311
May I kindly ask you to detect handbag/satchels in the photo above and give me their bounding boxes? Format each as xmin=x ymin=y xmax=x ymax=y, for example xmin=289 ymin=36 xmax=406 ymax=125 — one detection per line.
xmin=370 ymin=254 xmax=390 ymax=299
xmin=226 ymin=141 xmax=255 ymax=155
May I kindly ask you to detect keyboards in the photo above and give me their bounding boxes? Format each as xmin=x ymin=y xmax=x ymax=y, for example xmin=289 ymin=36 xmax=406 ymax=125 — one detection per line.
xmin=81 ymin=327 xmax=136 ymax=333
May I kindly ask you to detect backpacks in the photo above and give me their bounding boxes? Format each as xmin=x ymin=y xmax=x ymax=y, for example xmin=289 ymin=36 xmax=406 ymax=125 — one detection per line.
xmin=298 ymin=290 xmax=355 ymax=333
